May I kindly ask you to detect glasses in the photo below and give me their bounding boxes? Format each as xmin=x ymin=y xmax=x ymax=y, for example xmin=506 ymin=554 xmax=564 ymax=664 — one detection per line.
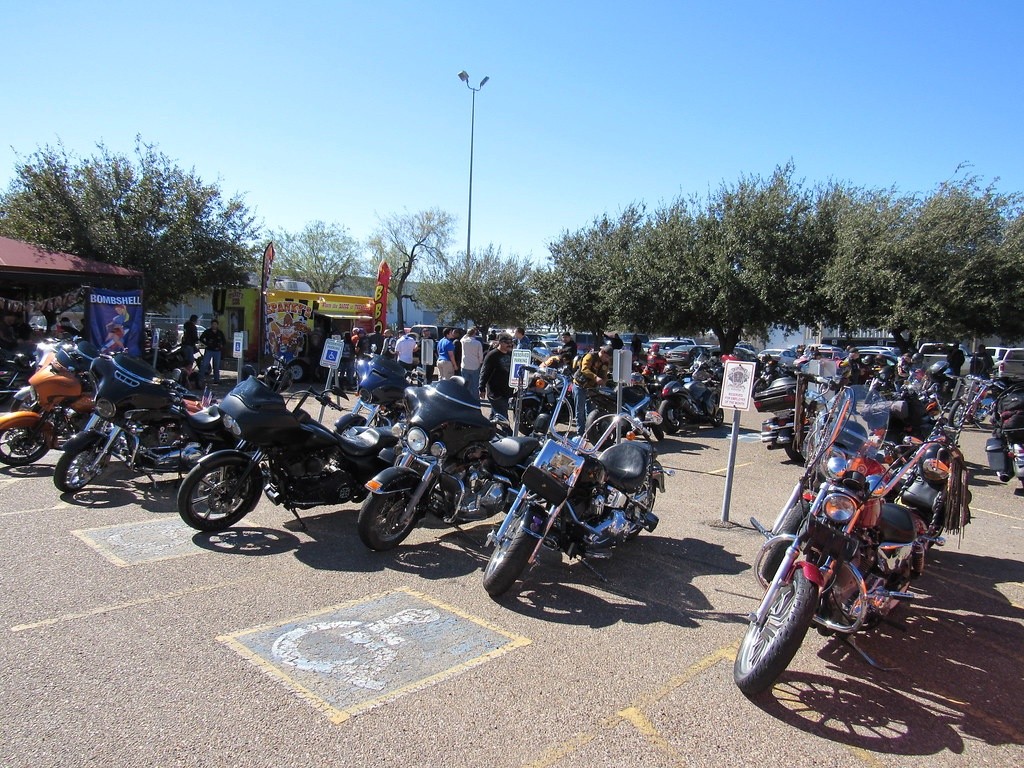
xmin=501 ymin=339 xmax=511 ymax=344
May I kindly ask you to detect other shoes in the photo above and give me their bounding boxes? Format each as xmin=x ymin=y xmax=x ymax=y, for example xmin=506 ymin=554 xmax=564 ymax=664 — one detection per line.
xmin=213 ymin=380 xmax=222 ymax=385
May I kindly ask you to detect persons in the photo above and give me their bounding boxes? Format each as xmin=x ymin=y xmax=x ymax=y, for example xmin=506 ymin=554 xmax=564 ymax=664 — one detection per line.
xmin=839 ymin=345 xmax=924 ymax=385
xmin=573 ymin=345 xmax=613 ymax=435
xmin=946 ymin=342 xmax=965 ymax=377
xmin=395 ymin=330 xmax=418 ymax=370
xmin=380 ymin=329 xmax=396 ymax=361
xmin=61 ymin=317 xmax=86 ymax=337
xmin=181 ymin=315 xmax=201 ymax=389
xmin=197 ymin=319 xmax=226 ymax=386
xmin=309 ymin=327 xmax=369 ymax=391
xmin=601 ymin=330 xmax=623 ymax=349
xmin=0 ymin=312 xmax=32 ymax=347
xmin=539 ymin=332 xmax=577 ymax=369
xmin=415 ymin=329 xmax=437 ymax=384
xmin=370 ymin=325 xmax=384 ymax=356
xmin=437 ymin=328 xmax=458 ymax=382
xmin=970 ymin=344 xmax=994 ymax=380
xmin=452 ymin=327 xmax=483 ymax=395
xmin=478 ymin=327 xmax=532 ymax=437
xmin=797 ymin=344 xmax=818 ymax=358
xmin=630 ymin=334 xmax=641 ymax=353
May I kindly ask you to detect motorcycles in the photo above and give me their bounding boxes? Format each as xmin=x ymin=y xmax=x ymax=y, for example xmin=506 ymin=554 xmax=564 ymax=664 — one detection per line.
xmin=0 ymin=324 xmax=1024 ymax=600
xmin=732 ymin=385 xmax=964 ymax=696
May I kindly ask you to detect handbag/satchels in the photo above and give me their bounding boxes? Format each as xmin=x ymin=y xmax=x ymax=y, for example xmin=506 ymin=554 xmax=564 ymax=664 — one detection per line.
xmin=1000 ymin=395 xmax=1024 ymax=429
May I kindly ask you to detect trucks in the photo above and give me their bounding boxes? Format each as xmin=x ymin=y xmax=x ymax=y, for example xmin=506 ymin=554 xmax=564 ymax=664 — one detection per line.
xmin=212 ymin=286 xmax=377 ymax=385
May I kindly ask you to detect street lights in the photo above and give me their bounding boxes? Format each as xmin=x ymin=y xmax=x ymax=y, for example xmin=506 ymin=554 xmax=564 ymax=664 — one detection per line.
xmin=457 ymin=69 xmax=491 ymax=333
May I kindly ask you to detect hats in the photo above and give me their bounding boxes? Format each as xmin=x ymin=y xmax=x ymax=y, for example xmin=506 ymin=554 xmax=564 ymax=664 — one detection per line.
xmin=357 ymin=328 xmax=365 ymax=334
xmin=849 ymin=349 xmax=857 ymax=353
xmin=600 ymin=345 xmax=614 ymax=356
